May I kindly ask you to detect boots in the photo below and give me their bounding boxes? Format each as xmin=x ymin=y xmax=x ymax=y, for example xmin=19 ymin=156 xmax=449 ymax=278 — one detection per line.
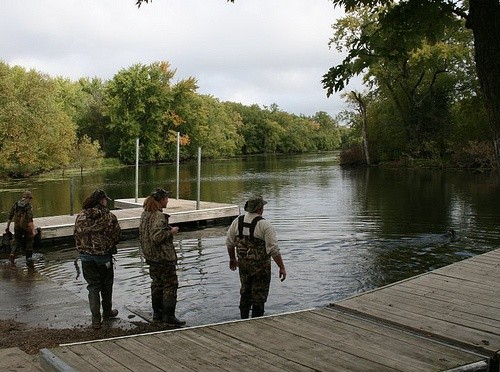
xmin=89 ymin=295 xmax=101 ymax=328
xmin=151 ymin=296 xmax=164 ymax=322
xmin=252 ymin=307 xmax=264 ymax=318
xmin=101 ymin=300 xmax=118 ymax=317
xmin=239 ymin=306 xmax=251 ymax=319
xmin=162 ymin=304 xmax=186 ymax=327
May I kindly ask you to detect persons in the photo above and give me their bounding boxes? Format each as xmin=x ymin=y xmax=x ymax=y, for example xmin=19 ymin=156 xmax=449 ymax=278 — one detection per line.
xmin=5 ymin=191 xmax=36 ymax=267
xmin=225 ymin=194 xmax=286 ymax=319
xmin=139 ymin=187 xmax=186 ymax=326
xmin=74 ymin=189 xmax=124 ymax=330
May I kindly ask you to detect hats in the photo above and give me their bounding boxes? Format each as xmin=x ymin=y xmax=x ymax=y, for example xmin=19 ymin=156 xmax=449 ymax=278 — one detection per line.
xmin=151 ymin=186 xmax=173 ymax=202
xmin=93 ymin=189 xmax=113 ymax=202
xmin=244 ymin=195 xmax=268 ymax=212
xmin=23 ymin=191 xmax=34 ymax=199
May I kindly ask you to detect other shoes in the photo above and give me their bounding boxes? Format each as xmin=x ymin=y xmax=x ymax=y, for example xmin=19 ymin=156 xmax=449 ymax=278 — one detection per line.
xmin=2 ymin=230 xmax=14 ymax=246
xmin=9 ymin=255 xmax=15 ymax=261
